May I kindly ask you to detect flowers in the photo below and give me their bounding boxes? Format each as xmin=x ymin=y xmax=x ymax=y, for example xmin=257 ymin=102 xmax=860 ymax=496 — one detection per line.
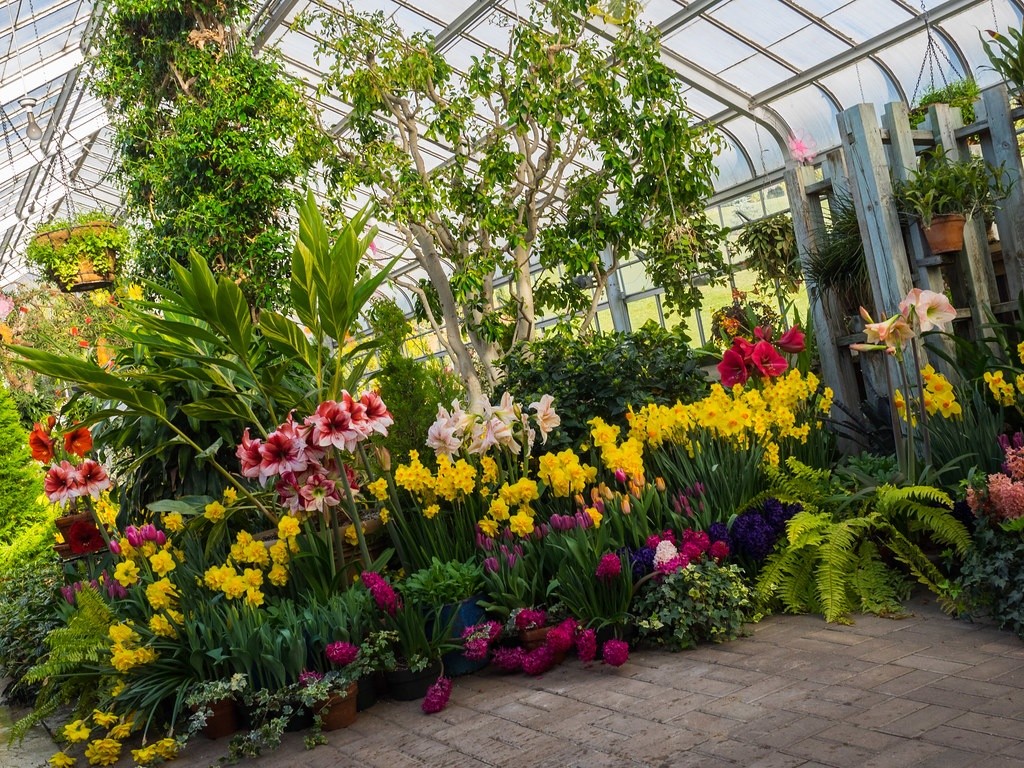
xmin=0 ymin=287 xmax=1024 ymax=766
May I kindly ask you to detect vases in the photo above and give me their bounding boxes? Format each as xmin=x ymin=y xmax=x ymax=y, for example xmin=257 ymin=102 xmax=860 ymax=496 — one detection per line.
xmin=393 ymin=662 xmax=435 ymax=702
xmin=523 ymin=625 xmax=564 ymax=665
xmin=312 ymin=680 xmax=358 ymax=731
xmin=54 ymin=508 xmax=97 ymax=556
xmin=191 ymin=696 xmax=233 ymax=740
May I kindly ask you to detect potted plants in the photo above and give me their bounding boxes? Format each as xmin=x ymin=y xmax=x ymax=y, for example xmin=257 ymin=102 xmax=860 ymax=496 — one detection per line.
xmin=891 ymin=141 xmax=1021 ymax=257
xmin=22 ymin=211 xmax=131 ymax=290
